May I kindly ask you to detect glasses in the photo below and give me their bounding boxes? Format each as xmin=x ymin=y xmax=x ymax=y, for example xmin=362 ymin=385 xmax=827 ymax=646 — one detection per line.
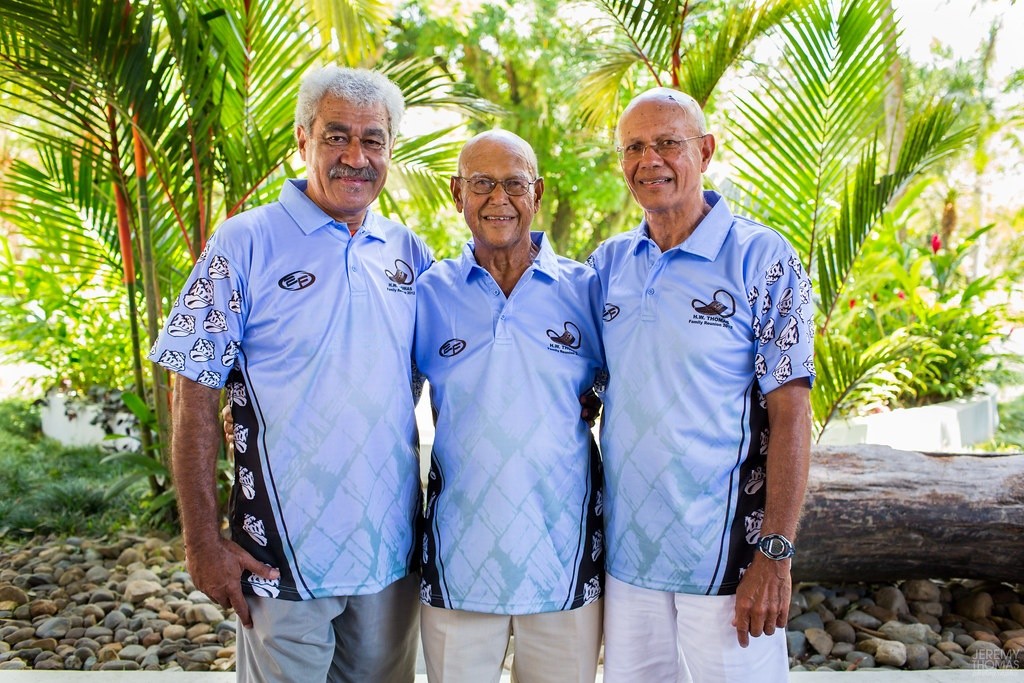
xmin=459 ymin=176 xmax=537 ymax=196
xmin=617 ymin=135 xmax=707 ymax=161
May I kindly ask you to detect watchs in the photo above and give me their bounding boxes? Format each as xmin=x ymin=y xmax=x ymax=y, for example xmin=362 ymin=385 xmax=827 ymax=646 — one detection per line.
xmin=756 ymin=534 xmax=795 ymax=560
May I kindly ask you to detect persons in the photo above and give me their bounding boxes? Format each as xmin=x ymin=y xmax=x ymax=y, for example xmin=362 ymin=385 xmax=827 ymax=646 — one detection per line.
xmin=148 ymin=67 xmax=602 ymax=683
xmin=581 ymin=86 xmax=815 ymax=683
xmin=412 ymin=129 xmax=608 ymax=683
